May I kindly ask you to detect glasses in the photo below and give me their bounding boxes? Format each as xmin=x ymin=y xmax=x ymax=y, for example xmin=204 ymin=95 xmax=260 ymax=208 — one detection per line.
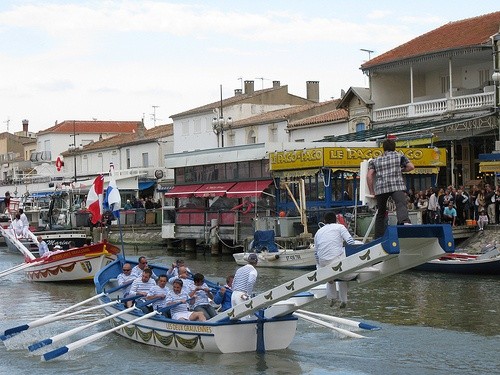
xmin=123 ymin=269 xmax=129 ymax=271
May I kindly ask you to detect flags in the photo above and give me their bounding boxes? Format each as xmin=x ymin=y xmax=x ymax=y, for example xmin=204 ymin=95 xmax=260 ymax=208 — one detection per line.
xmin=104 ymin=169 xmax=121 ymax=221
xmin=86 ymin=175 xmax=103 ymax=225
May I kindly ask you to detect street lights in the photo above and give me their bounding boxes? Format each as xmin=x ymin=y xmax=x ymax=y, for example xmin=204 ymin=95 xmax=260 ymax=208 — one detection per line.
xmin=212 ymin=115 xmax=233 ymax=147
xmin=68 ymin=142 xmax=83 ymax=183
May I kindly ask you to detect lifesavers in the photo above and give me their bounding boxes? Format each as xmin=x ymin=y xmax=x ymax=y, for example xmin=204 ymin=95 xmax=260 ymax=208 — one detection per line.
xmin=55 ymin=156 xmax=62 ymax=172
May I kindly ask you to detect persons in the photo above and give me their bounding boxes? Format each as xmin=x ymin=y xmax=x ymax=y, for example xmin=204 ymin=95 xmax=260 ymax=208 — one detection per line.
xmin=146 ymin=274 xmax=173 ymax=318
xmin=366 ymin=139 xmax=414 ymax=240
xmin=124 ymin=196 xmax=163 ymax=209
xmin=128 ymin=269 xmax=157 ymax=296
xmin=4 ymin=192 xmax=12 ymax=214
xmin=231 ymin=254 xmax=258 ymax=321
xmin=166 ymin=279 xmax=206 ymax=321
xmin=314 ymin=212 xmax=363 ymax=309
xmin=130 ymin=256 xmax=158 ymax=283
xmin=214 ymin=275 xmax=237 ymax=312
xmin=166 ymin=258 xmax=195 ymax=293
xmin=117 ymin=261 xmax=139 ymax=296
xmin=37 ymin=236 xmax=49 ymax=257
xmin=186 ymin=273 xmax=217 ymax=320
xmin=402 ymin=184 xmax=500 ymax=231
xmin=11 ymin=209 xmax=29 ymax=239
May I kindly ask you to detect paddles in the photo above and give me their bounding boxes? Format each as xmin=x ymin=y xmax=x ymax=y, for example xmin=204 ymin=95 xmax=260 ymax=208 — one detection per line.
xmin=27 ymin=297 xmax=161 ymax=353
xmin=292 ymin=309 xmax=383 ymax=339
xmin=40 ymin=296 xmax=196 ymax=364
xmin=0 ymin=280 xmax=144 ymax=342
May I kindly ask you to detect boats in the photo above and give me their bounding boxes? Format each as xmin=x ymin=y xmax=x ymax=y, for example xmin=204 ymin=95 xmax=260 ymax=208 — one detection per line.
xmin=94 ymin=223 xmax=454 ymax=355
xmin=232 ymin=229 xmax=500 ymax=276
xmin=0 ymin=214 xmax=121 ymax=284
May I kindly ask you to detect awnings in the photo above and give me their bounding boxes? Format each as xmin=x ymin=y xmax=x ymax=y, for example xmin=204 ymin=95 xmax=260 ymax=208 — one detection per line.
xmin=31 ymin=182 xmax=155 ymax=197
xmin=165 ymin=180 xmax=273 ymax=197
xmin=312 ymin=113 xmax=500 ymax=147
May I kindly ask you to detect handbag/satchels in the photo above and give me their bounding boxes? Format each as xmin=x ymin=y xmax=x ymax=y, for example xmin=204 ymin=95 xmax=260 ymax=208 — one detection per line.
xmin=475 ymin=198 xmax=479 ymax=205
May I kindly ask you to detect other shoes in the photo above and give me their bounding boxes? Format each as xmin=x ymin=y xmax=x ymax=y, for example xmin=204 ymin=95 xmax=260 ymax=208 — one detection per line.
xmin=330 ymin=299 xmax=336 ymax=307
xmin=479 ymin=228 xmax=481 ymax=230
xmin=482 ymin=228 xmax=483 ymax=231
xmin=340 ymin=302 xmax=346 ymax=309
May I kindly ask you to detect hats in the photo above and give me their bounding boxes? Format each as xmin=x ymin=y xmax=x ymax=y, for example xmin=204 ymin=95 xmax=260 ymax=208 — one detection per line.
xmin=54 ymin=245 xmax=60 ymax=248
xmin=248 ymin=254 xmax=257 ymax=260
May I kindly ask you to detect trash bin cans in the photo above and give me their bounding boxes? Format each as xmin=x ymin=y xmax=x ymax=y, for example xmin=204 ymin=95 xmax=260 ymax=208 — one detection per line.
xmin=268 ymin=217 xmax=280 ymax=235
xmin=120 ymin=208 xmax=162 ymax=225
xmin=255 ymin=217 xmax=267 ymax=233
xmin=278 ymin=215 xmax=309 ymax=238
xmin=357 ymin=212 xmax=376 ymax=236
